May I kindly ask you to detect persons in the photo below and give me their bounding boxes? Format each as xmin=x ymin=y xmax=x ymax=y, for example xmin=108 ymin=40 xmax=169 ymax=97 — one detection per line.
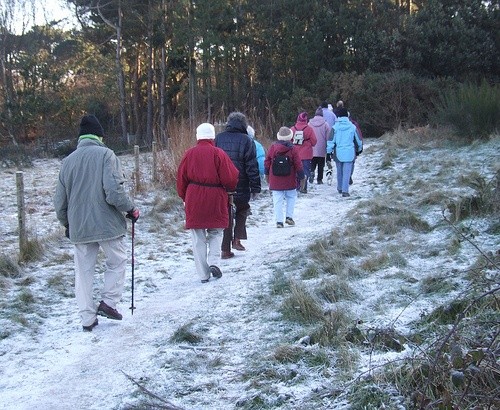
xmin=318 ymin=101 xmax=362 ymax=184
xmin=306 ymin=108 xmax=330 ymax=185
xmin=54 ymin=113 xmax=141 ymax=331
xmin=212 ymin=112 xmax=261 ymax=259
xmin=326 ymin=106 xmax=362 ymax=197
xmin=176 ymin=122 xmax=241 ymax=284
xmin=243 ymin=123 xmax=266 ymax=184
xmin=288 ymin=112 xmax=316 ymax=193
xmin=265 ymin=126 xmax=306 ymax=228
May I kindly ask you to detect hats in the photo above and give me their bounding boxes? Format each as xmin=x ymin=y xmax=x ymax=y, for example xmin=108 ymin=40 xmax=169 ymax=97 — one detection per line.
xmin=277 ymin=126 xmax=293 ymax=141
xmin=196 ymin=123 xmax=215 ymax=140
xmin=298 ymin=112 xmax=307 ymax=123
xmin=78 ymin=115 xmax=104 ymax=138
xmin=315 ymin=107 xmax=324 ymax=118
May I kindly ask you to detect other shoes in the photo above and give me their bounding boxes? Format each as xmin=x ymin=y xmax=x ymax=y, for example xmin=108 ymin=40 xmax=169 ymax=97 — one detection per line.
xmin=338 ymin=190 xmax=350 ymax=197
xmin=285 ymin=218 xmax=295 ymax=225
xmin=277 ymin=222 xmax=284 ymax=228
xmin=201 ymin=279 xmax=209 ymax=283
xmin=221 ymin=252 xmax=234 ymax=259
xmin=209 ymin=265 xmax=222 ymax=279
xmin=232 ymin=240 xmax=246 ymax=250
xmin=317 ymin=180 xmax=323 ymax=184
xmin=309 ymin=176 xmax=314 ymax=183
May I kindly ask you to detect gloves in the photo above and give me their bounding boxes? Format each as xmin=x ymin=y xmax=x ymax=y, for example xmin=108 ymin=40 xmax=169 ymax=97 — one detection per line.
xmin=64 ymin=224 xmax=69 ymax=238
xmin=327 ymin=153 xmax=333 ymax=162
xmin=126 ymin=207 xmax=140 ymax=222
xmin=299 ymin=179 xmax=304 ymax=191
xmin=265 ymin=175 xmax=269 ymax=183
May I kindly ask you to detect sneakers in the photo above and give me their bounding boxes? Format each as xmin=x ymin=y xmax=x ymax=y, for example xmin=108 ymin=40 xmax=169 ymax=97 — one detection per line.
xmin=98 ymin=300 xmax=123 ymax=320
xmin=300 ymin=189 xmax=308 ymax=193
xmin=82 ymin=318 xmax=98 ymax=331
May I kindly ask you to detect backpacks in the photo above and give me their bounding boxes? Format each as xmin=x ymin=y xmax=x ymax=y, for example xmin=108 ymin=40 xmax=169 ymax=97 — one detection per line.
xmin=272 ymin=153 xmax=290 ymax=175
xmin=292 ymin=124 xmax=307 ymax=145
